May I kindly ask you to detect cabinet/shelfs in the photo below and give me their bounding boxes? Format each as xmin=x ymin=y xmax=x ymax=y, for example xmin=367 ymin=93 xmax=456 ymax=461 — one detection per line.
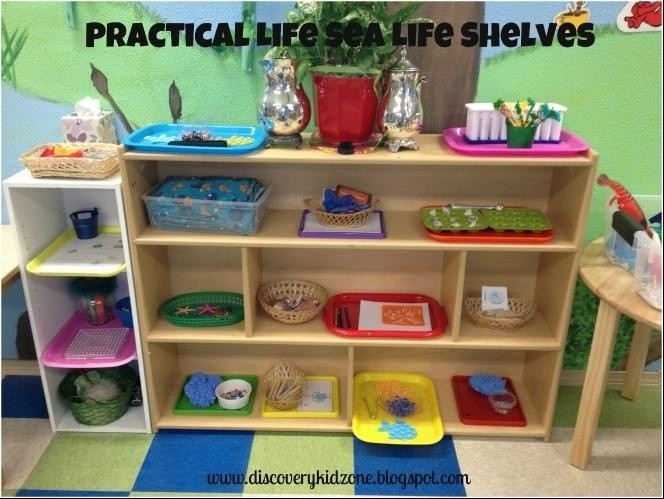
xmin=3 ymin=128 xmax=602 ymax=446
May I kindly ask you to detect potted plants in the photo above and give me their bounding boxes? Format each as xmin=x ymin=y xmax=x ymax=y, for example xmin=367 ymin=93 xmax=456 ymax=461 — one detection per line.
xmin=259 ymin=1 xmax=422 ymax=143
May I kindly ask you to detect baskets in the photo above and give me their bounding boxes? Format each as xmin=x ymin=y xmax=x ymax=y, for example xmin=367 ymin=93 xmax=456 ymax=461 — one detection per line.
xmin=260 ymin=362 xmax=307 ymax=409
xmin=257 ymin=280 xmax=328 ymax=324
xmin=58 ymin=368 xmax=136 ymax=426
xmin=465 ymin=296 xmax=538 ymax=332
xmin=18 ymin=143 xmax=120 ymax=179
xmin=303 ymin=197 xmax=381 ymax=228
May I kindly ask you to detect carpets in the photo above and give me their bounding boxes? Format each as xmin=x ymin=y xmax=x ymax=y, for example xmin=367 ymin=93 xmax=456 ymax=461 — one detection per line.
xmin=4 ymin=423 xmax=468 ymax=498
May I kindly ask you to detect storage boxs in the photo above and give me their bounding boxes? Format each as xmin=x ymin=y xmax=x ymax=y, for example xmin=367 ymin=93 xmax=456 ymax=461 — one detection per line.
xmin=604 ymin=194 xmax=663 ymax=312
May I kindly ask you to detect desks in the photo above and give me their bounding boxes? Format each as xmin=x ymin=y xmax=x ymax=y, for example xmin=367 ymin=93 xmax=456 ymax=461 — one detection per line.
xmin=569 ymin=233 xmax=663 ymax=476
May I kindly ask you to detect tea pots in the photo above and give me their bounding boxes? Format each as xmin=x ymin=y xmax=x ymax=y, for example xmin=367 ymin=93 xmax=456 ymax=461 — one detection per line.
xmin=258 ymin=56 xmax=312 ymax=150
xmin=378 ymin=44 xmax=429 ymax=153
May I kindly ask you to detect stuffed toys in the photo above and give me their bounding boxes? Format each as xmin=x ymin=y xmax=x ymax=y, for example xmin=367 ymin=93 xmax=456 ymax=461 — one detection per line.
xmin=597 ymin=174 xmax=653 ymax=240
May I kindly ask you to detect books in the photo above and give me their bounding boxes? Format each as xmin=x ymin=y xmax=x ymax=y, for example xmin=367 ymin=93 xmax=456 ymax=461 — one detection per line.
xmin=358 ymin=300 xmax=432 ymax=331
